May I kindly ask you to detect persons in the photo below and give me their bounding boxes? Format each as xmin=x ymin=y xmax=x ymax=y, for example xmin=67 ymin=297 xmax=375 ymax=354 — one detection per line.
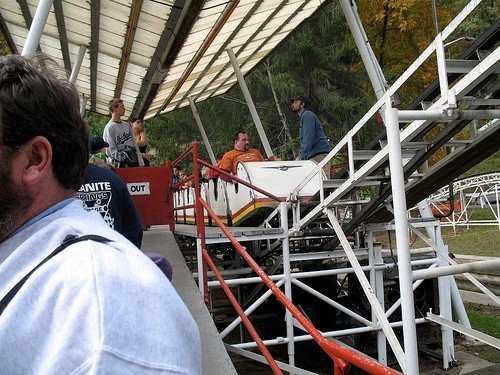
xmin=192 ymin=152 xmax=225 ymax=201
xmin=219 ymin=130 xmax=279 ymax=184
xmin=126 ymin=118 xmax=156 ymax=167
xmin=0 ymin=55 xmax=203 ymax=375
xmin=102 ymin=98 xmax=150 ymax=231
xmin=292 ymin=95 xmax=331 ymax=202
xmin=173 ymin=166 xmax=192 ymax=193
xmin=76 ymin=137 xmax=173 ymax=282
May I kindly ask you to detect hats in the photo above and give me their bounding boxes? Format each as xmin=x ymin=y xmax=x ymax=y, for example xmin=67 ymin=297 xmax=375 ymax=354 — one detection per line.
xmin=89 ymin=137 xmax=109 ymax=150
xmin=290 ymin=96 xmax=311 ymax=105
